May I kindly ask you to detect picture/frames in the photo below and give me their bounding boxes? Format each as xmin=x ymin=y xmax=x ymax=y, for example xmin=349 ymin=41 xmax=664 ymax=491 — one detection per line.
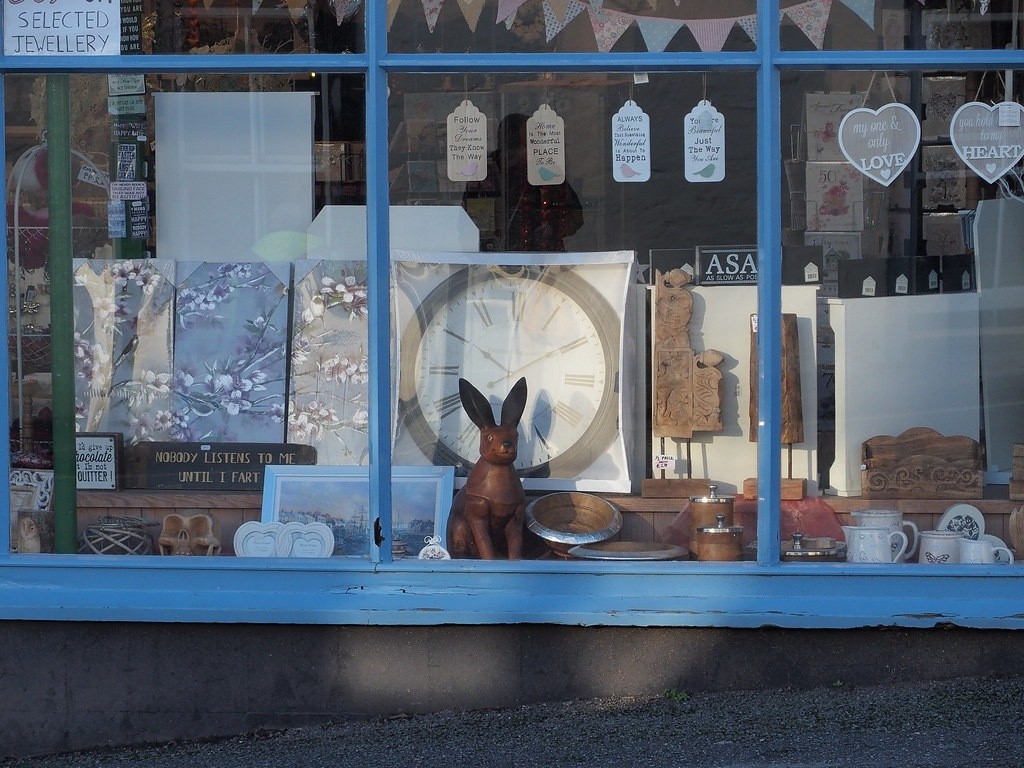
xmin=262 ymin=463 xmax=455 ymax=562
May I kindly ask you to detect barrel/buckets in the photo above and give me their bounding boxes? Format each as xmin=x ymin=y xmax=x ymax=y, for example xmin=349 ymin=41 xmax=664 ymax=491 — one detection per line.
xmin=784 ymin=531 xmax=845 ymax=562
xmin=686 ymin=484 xmax=735 ymax=561
xmin=696 ymin=514 xmax=743 ymax=561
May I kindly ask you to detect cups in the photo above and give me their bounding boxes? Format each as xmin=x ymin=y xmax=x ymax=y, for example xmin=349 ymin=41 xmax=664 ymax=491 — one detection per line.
xmin=839 ymin=525 xmax=907 ymax=564
xmin=851 ymin=509 xmax=919 ymax=564
xmin=916 ymin=528 xmax=963 ymax=564
xmin=955 ymin=537 xmax=1015 ymax=563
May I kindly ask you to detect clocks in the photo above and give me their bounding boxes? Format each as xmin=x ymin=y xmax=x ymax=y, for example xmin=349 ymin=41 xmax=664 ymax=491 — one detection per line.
xmin=396 ymin=261 xmax=624 ymax=480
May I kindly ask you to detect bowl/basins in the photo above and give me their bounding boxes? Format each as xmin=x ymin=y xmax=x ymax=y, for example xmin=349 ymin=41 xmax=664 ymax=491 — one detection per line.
xmin=568 ymin=540 xmax=690 ymax=563
xmin=528 ymin=490 xmax=623 ymax=557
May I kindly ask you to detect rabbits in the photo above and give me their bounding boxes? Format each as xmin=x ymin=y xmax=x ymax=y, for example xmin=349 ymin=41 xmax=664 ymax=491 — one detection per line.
xmin=447 ymin=374 xmax=532 ymax=561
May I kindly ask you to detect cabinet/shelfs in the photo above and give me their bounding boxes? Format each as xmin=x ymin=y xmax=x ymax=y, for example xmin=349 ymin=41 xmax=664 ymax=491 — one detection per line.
xmin=781 ymin=81 xmax=896 ymax=433
xmin=389 ymin=86 xmax=513 ymax=253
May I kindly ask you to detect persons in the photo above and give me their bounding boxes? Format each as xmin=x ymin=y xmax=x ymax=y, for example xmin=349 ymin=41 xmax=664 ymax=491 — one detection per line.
xmin=460 ymin=112 xmax=586 ymax=254
